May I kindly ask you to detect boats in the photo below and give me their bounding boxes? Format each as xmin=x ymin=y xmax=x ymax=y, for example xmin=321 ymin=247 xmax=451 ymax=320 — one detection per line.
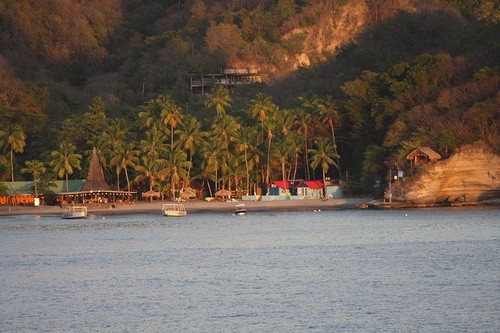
xmin=234 ymin=204 xmax=247 ymax=217
xmin=62 ymin=206 xmax=88 ymax=219
xmin=162 ymin=203 xmax=187 ymax=217
